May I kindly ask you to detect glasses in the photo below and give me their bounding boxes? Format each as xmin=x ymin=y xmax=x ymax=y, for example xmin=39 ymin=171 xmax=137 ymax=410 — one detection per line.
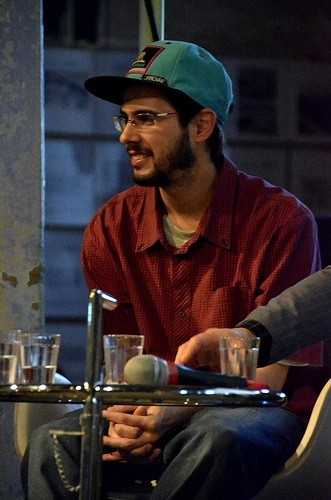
xmin=113 ymin=112 xmax=179 ymax=132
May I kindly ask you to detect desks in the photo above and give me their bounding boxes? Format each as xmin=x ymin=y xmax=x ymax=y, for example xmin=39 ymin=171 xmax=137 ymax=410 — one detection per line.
xmin=0 ymin=385 xmax=287 ymax=500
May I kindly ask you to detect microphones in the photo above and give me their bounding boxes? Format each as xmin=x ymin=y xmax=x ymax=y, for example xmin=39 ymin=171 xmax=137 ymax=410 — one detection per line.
xmin=123 ymin=354 xmax=269 ymax=391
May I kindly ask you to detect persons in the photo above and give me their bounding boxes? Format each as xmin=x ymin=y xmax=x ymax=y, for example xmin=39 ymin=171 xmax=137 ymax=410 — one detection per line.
xmin=21 ymin=40 xmax=324 ymax=500
xmin=174 ymin=265 xmax=331 ymax=378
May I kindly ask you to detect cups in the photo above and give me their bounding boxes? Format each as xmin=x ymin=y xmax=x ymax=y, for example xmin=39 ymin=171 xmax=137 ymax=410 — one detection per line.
xmin=0 ymin=330 xmax=21 ymax=385
xmin=103 ymin=335 xmax=144 ymax=385
xmin=220 ymin=336 xmax=260 ymax=380
xmin=20 ymin=333 xmax=60 ymax=384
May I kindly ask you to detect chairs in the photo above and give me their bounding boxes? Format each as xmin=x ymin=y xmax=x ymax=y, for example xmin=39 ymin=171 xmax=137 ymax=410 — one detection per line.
xmin=13 ymin=216 xmax=331 ymax=500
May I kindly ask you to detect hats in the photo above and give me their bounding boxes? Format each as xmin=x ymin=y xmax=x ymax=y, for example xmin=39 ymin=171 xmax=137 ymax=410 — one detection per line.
xmin=84 ymin=40 xmax=233 ymax=126
